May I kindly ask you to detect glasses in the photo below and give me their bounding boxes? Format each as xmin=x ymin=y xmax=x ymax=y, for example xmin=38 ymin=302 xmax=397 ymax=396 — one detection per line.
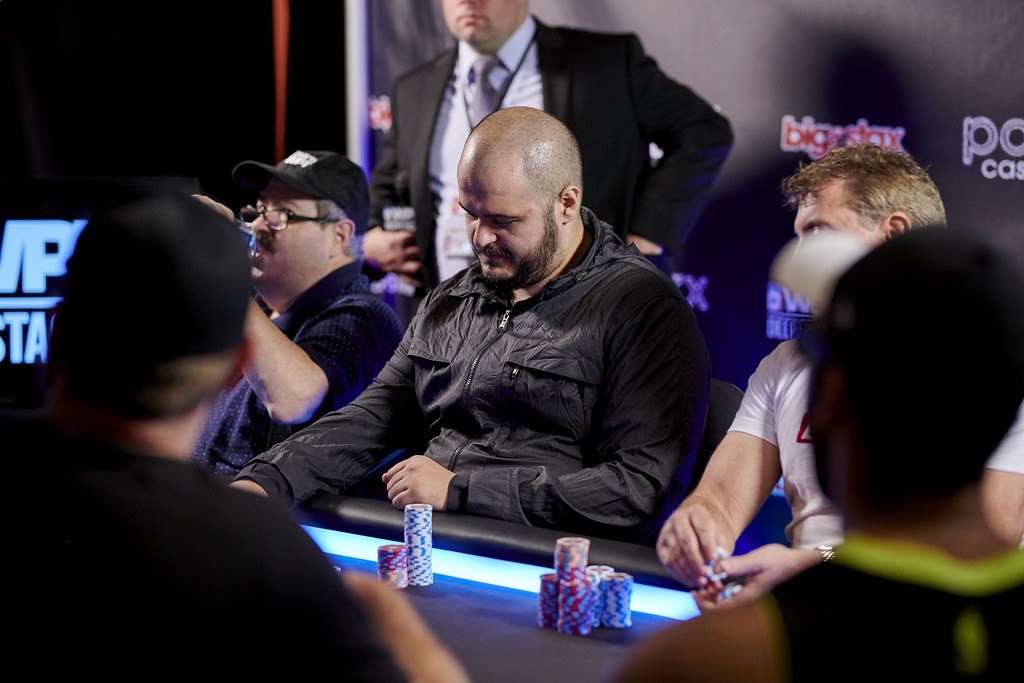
xmin=239 ymin=205 xmax=340 ymax=236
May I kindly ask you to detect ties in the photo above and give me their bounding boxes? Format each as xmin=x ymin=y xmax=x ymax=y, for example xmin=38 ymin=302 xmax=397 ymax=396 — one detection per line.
xmin=468 ymin=58 xmax=503 ymax=129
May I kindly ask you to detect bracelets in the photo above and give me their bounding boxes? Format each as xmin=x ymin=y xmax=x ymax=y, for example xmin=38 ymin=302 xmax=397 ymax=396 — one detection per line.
xmin=816 ymin=545 xmax=835 ymax=563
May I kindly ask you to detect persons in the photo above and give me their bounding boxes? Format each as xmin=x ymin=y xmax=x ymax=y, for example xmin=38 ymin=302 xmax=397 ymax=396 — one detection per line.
xmin=362 ymin=0 xmax=735 ymax=322
xmin=227 ymin=106 xmax=708 ymax=544
xmin=189 ymin=151 xmax=403 ymax=476
xmin=611 ymin=222 xmax=1023 ymax=683
xmin=657 ymin=142 xmax=1023 ymax=613
xmin=1 ymin=193 xmax=468 ymax=683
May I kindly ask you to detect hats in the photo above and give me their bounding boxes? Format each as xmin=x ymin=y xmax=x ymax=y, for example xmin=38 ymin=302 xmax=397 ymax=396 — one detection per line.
xmin=66 ymin=187 xmax=249 ymax=362
xmin=232 ymin=150 xmax=369 ymax=236
xmin=795 ymin=228 xmax=1023 ymax=495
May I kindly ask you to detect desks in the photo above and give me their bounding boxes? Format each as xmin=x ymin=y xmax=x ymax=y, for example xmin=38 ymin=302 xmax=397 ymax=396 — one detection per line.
xmin=283 ymin=492 xmax=705 ymax=683
